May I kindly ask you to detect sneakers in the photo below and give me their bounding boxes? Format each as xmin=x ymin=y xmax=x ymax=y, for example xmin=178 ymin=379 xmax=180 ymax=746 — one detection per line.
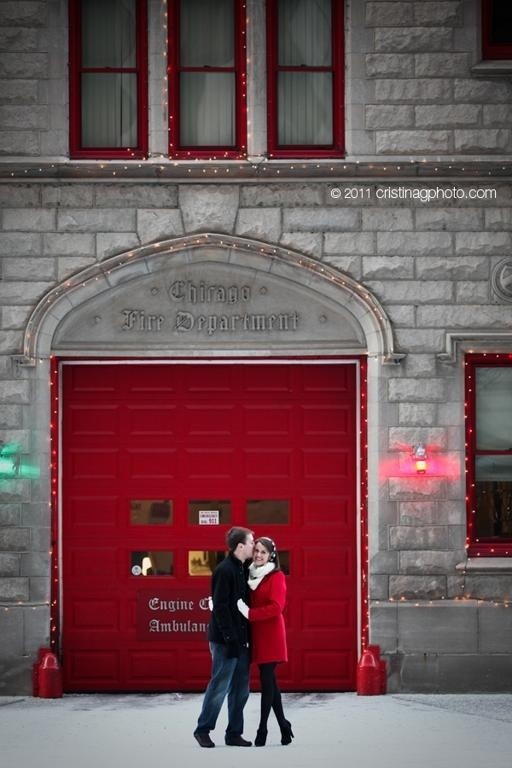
xmin=194 ymin=727 xmax=214 ymax=747
xmin=225 ymin=733 xmax=252 ymax=747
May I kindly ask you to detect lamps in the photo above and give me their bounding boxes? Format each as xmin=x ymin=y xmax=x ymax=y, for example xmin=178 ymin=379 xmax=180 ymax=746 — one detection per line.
xmin=411 ymin=440 xmax=428 ymax=475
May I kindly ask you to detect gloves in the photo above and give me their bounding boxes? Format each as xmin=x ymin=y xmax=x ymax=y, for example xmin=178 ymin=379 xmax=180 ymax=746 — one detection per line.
xmin=208 ymin=597 xmax=213 ymax=610
xmin=238 ymin=598 xmax=250 ymax=618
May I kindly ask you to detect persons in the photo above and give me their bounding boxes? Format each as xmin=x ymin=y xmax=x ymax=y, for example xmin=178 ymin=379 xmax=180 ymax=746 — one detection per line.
xmin=193 ymin=526 xmax=253 ymax=748
xmin=209 ymin=536 xmax=295 ymax=746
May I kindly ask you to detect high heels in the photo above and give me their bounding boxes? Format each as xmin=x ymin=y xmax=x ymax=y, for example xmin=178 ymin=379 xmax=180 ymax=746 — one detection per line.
xmin=280 ymin=721 xmax=294 ymax=745
xmin=254 ymin=728 xmax=267 ymax=746
